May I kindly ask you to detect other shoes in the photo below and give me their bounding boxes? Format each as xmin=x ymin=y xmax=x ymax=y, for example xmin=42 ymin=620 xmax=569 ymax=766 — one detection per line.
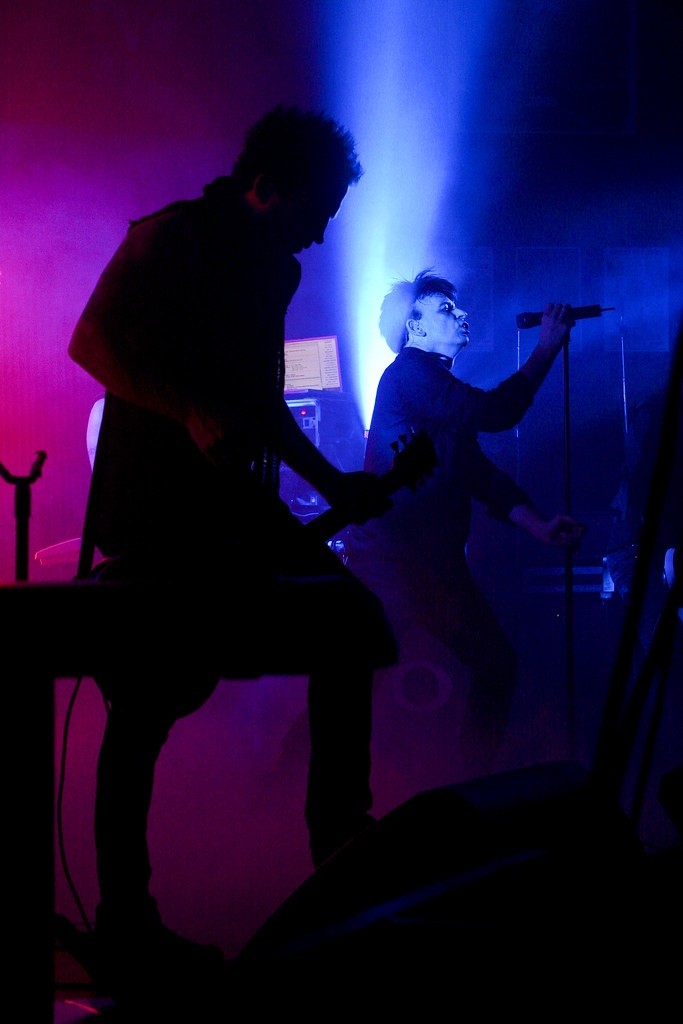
xmin=308 ymin=815 xmax=375 ymax=865
xmin=95 ymin=905 xmax=222 ymax=972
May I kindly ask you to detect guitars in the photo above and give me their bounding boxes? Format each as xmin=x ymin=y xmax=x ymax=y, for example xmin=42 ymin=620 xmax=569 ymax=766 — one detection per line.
xmin=305 ymin=429 xmax=438 ymax=543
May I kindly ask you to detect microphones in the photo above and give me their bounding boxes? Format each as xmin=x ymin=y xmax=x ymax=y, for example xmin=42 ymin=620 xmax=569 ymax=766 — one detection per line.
xmin=517 ymin=304 xmax=615 ymax=330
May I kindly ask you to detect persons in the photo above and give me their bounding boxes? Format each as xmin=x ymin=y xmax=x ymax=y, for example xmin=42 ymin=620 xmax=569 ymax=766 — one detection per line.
xmin=67 ymin=106 xmax=401 ymax=991
xmin=277 ymin=275 xmax=588 ymax=783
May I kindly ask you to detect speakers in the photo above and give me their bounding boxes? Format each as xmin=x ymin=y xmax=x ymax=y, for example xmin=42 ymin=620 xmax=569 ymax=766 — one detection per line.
xmin=205 ymin=758 xmax=683 ymax=1024
